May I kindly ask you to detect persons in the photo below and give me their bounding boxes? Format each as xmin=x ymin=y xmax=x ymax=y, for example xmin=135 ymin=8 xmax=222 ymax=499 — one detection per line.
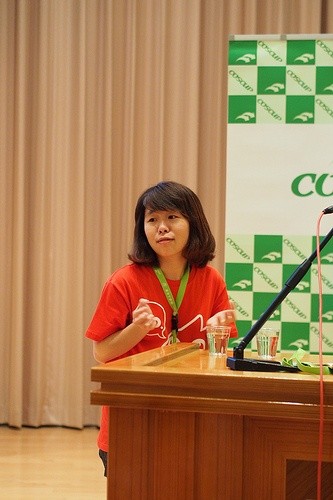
xmin=84 ymin=180 xmax=238 ymax=479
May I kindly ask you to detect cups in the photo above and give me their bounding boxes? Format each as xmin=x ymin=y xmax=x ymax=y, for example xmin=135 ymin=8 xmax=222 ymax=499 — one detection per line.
xmin=256 ymin=328 xmax=280 ymax=358
xmin=206 ymin=326 xmax=232 ymax=356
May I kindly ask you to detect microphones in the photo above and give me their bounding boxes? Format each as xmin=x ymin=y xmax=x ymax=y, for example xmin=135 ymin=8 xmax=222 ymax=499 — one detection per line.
xmin=322 ymin=205 xmax=333 ymax=214
